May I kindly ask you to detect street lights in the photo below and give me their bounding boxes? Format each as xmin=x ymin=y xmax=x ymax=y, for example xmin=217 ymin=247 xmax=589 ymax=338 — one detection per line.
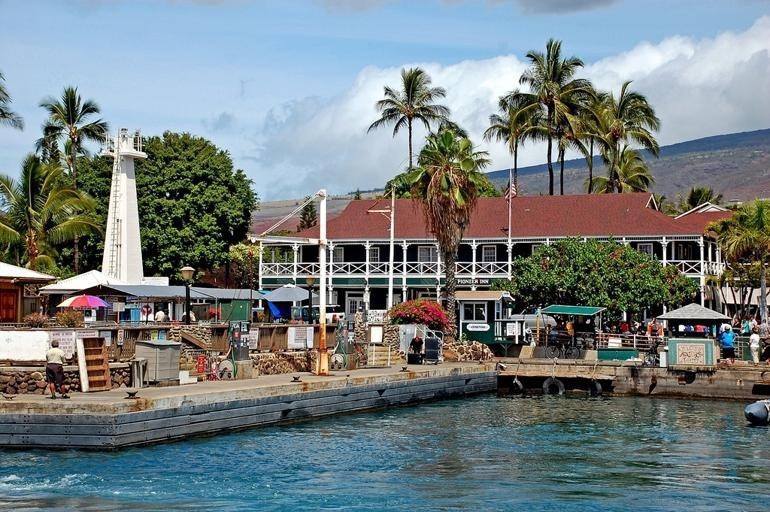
xmin=180 ymin=262 xmax=197 ymax=324
xmin=306 ymin=272 xmax=316 ymax=325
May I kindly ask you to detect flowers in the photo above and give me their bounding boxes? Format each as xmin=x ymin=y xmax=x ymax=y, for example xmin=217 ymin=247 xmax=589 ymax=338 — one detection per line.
xmin=382 ymin=298 xmax=454 ymax=334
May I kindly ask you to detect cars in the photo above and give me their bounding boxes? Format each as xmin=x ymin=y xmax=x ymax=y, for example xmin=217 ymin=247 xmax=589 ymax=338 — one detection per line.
xmin=304 ymin=304 xmax=346 ymax=323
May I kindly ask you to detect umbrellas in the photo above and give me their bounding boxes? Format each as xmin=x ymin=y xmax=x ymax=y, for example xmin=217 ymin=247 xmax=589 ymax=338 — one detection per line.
xmin=56 ymin=294 xmax=111 ymax=328
xmin=263 ymin=283 xmax=318 ymax=320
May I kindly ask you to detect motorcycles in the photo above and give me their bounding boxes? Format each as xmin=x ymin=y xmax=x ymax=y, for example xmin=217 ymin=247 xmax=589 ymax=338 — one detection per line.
xmin=643 ymin=332 xmax=669 ymax=367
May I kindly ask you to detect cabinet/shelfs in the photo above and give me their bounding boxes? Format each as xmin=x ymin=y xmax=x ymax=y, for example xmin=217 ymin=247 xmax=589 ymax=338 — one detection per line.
xmin=77 ymin=336 xmax=113 ymax=391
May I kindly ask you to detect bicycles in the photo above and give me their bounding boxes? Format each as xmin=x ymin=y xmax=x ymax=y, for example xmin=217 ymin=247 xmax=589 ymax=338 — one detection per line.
xmin=544 ymin=336 xmax=581 ymax=359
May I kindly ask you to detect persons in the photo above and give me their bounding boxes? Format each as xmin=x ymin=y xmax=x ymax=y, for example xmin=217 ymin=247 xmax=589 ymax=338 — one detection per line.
xmin=45 ymin=340 xmax=70 ymax=399
xmin=408 ymin=336 xmax=423 ymax=354
xmin=155 ymin=307 xmax=164 ymax=322
xmin=180 ymin=306 xmax=196 ymax=321
xmin=603 ymin=318 xmax=664 ymax=336
xmin=199 ymin=307 xmax=210 ymax=320
xmin=161 ymin=312 xmax=170 ymax=322
xmin=679 ymin=312 xmax=770 ymax=368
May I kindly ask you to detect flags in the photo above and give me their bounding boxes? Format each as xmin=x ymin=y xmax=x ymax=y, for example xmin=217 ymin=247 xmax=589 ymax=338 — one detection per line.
xmin=504 ymin=173 xmax=517 ymax=204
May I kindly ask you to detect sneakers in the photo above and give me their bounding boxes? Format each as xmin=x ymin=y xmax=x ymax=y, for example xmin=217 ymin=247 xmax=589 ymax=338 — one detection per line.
xmin=51 ymin=394 xmax=70 ymax=399
